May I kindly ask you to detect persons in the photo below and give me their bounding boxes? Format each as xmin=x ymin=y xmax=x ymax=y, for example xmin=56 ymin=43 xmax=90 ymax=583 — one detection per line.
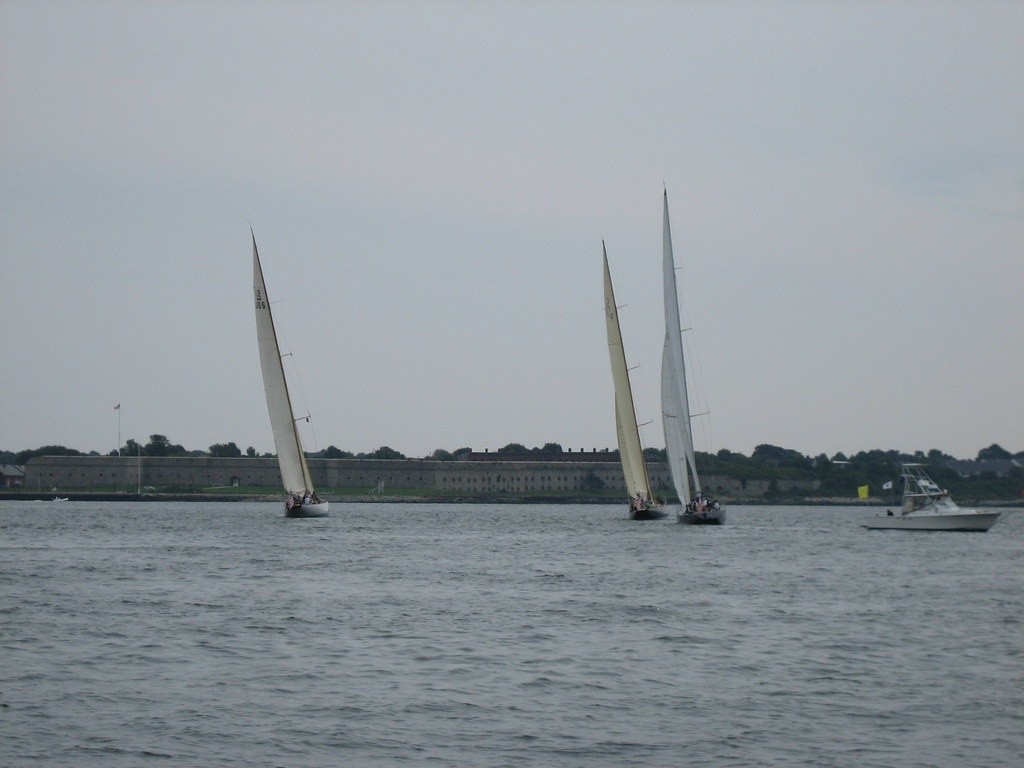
xmin=685 ymin=497 xmax=719 ymax=514
xmin=293 ymin=491 xmax=321 ymax=504
xmin=630 ymin=497 xmax=653 ymax=513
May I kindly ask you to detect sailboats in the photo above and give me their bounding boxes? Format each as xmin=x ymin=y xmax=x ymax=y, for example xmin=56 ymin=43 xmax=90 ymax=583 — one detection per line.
xmin=661 ymin=186 xmax=727 ymax=525
xmin=601 ymin=239 xmax=669 ymax=521
xmin=249 ymin=225 xmax=329 ymax=519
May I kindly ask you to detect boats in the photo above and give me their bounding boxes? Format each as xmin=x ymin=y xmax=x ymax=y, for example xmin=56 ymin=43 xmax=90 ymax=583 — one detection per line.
xmin=53 ymin=496 xmax=68 ymax=502
xmin=864 ymin=461 xmax=1001 ymax=533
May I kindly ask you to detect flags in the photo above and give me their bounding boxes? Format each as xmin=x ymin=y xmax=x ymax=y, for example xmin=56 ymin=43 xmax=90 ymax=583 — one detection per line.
xmin=882 ymin=481 xmax=892 ymax=491
xmin=634 ymin=498 xmax=640 ymax=510
xmin=858 ymin=485 xmax=869 ymax=499
xmin=114 ymin=404 xmax=120 ymax=409
xmin=287 ymin=492 xmax=294 ymax=510
xmin=696 ymin=500 xmax=703 ymax=513
